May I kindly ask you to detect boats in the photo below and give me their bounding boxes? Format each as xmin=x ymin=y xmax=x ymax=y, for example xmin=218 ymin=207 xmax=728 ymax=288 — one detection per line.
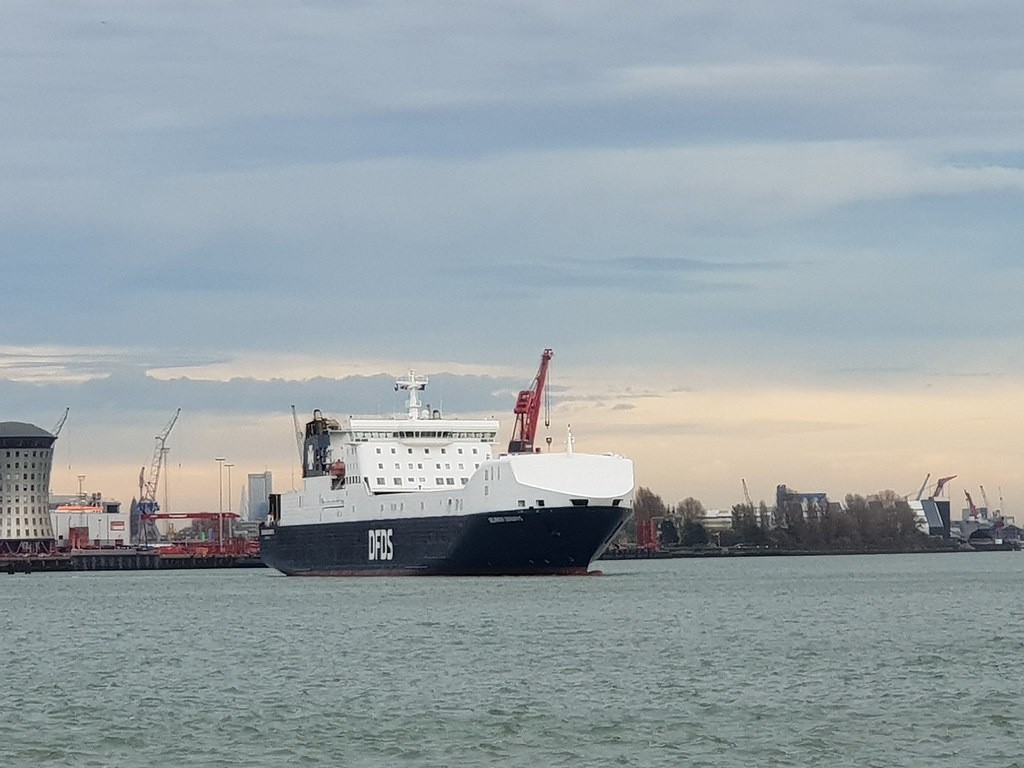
xmin=967 ymin=523 xmax=1021 ymax=551
xmin=256 ymin=347 xmax=634 ymax=579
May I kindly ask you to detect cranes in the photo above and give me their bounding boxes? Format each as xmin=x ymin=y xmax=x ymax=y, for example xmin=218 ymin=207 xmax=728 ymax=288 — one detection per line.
xmin=49 ymin=404 xmax=312 ymax=554
xmin=915 ymin=471 xmax=1008 ymax=529
